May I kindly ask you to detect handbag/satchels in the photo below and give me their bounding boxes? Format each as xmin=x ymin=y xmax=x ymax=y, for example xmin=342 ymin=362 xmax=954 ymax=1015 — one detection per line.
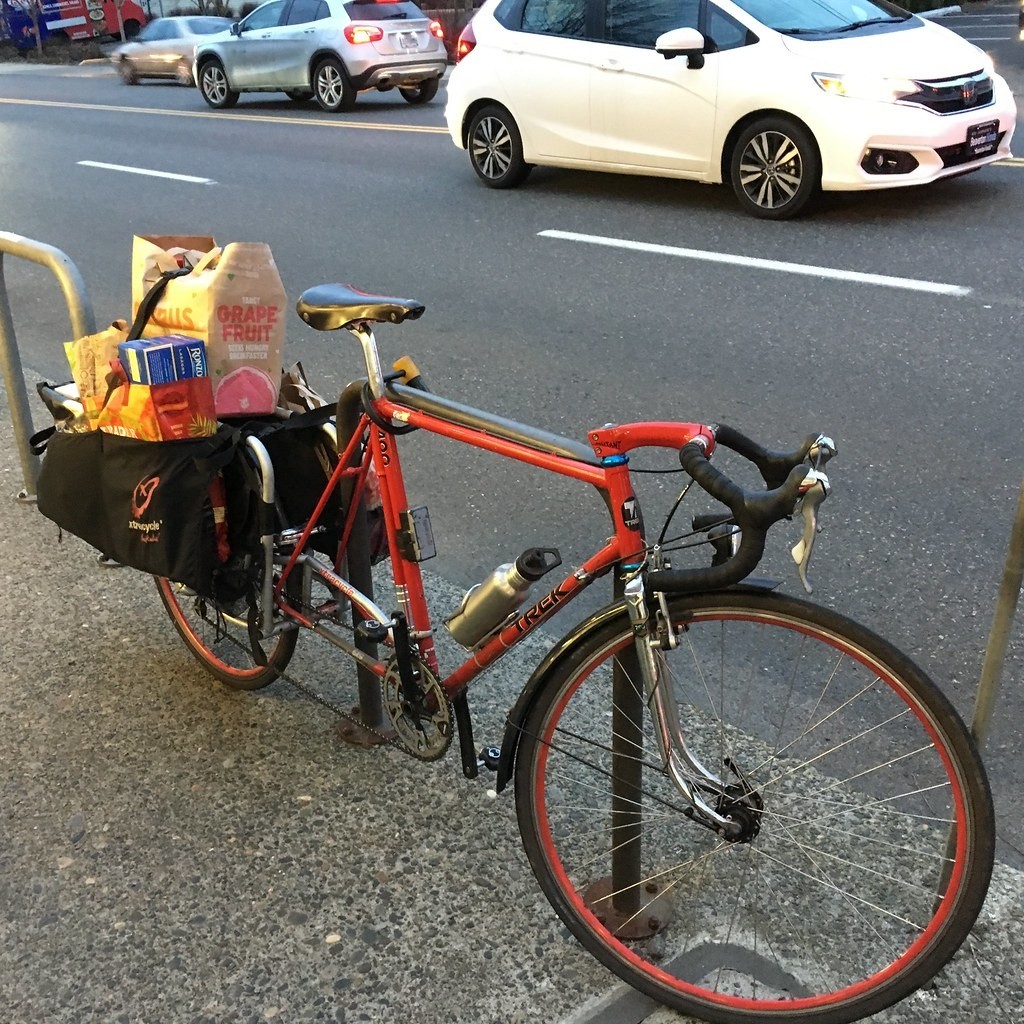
xmin=253 ymin=417 xmax=388 ymax=566
xmin=131 ymin=233 xmax=287 ymax=415
xmin=63 ymin=319 xmax=215 ymax=439
xmin=28 ymin=423 xmax=255 ymax=605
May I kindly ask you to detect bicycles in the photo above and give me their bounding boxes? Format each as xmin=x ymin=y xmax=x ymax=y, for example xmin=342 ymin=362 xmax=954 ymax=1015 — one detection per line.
xmin=153 ymin=284 xmax=997 ymax=1023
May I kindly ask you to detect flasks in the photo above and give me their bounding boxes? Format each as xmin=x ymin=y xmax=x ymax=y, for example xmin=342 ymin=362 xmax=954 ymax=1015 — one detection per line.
xmin=443 ymin=547 xmax=563 ymax=648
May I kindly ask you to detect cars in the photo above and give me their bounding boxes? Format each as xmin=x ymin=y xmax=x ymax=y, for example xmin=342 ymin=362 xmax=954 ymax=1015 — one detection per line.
xmin=192 ymin=0 xmax=448 ymax=110
xmin=109 ymin=16 xmax=236 ymax=89
xmin=442 ymin=0 xmax=1019 ymax=221
